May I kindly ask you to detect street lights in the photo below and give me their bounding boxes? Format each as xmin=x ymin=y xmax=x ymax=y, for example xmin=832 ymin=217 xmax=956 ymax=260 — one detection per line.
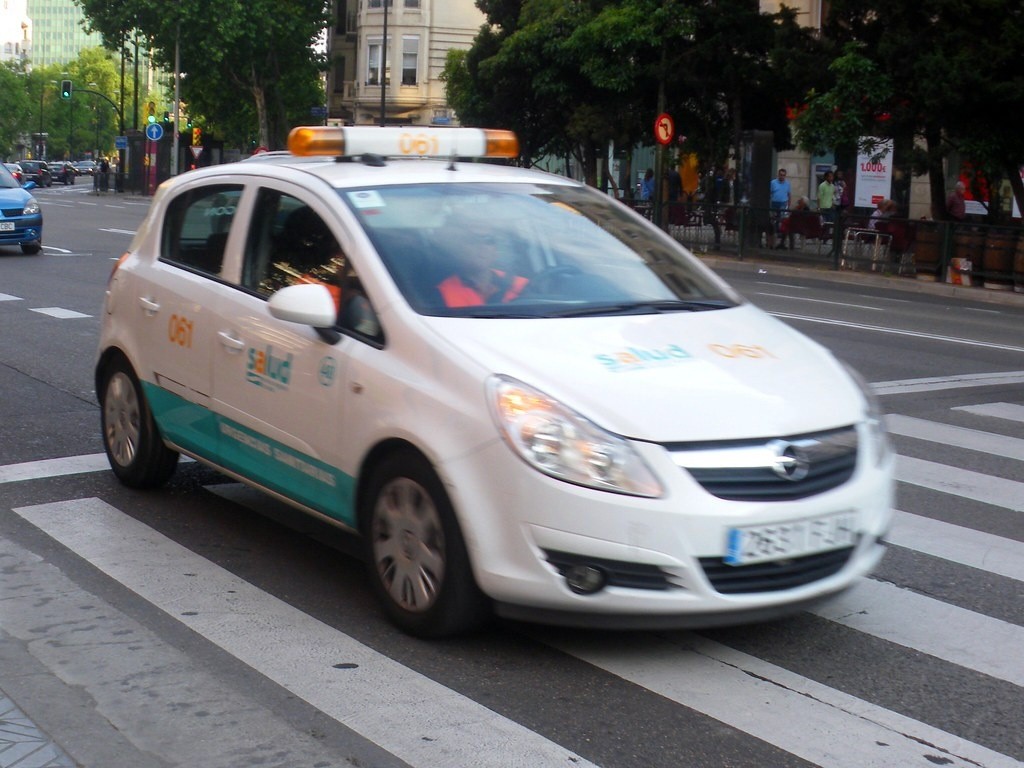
xmin=729 ymin=76 xmax=762 ymax=207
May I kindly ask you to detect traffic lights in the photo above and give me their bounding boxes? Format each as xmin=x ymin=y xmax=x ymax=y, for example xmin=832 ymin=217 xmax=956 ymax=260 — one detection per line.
xmin=61 ymin=80 xmax=72 ymax=99
xmin=190 ymin=164 xmax=197 ymax=169
xmin=187 ymin=118 xmax=192 ymax=128
xmin=252 ymin=140 xmax=256 ymax=144
xmin=147 ymin=102 xmax=156 ymax=123
xmin=597 ymin=176 xmax=602 ymax=188
xmin=164 ymin=111 xmax=169 ymax=123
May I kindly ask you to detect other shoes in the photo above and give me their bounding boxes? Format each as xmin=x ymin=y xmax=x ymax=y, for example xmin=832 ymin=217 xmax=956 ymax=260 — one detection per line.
xmin=777 ymin=243 xmax=785 ymax=248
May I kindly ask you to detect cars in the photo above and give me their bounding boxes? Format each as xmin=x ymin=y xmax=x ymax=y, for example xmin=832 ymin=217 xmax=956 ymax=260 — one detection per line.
xmin=48 ymin=163 xmax=76 ymax=185
xmin=93 ymin=125 xmax=897 ymax=641
xmin=0 ymin=163 xmax=25 ymax=186
xmin=0 ymin=162 xmax=43 ymax=255
xmin=15 ymin=160 xmax=52 ymax=188
xmin=76 ymin=161 xmax=96 ymax=176
xmin=91 ymin=166 xmax=112 ymax=175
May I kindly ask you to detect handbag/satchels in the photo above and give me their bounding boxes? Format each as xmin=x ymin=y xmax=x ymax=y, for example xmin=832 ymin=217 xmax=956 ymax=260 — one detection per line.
xmin=649 ymin=191 xmax=654 ymax=199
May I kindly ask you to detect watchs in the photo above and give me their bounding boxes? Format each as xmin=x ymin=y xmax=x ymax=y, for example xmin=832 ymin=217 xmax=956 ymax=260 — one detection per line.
xmin=817 ymin=208 xmax=821 ymax=210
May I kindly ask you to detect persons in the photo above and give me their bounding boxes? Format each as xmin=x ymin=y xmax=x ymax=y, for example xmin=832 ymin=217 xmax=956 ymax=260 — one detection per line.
xmin=1000 ymin=186 xmax=1013 ymax=211
xmin=669 ymin=163 xmax=735 ymax=204
xmin=868 ymin=199 xmax=915 ymax=253
xmin=770 ymin=168 xmax=792 ymax=216
xmin=946 ymin=181 xmax=966 ymax=220
xmin=816 ymin=169 xmax=851 ymax=246
xmin=428 ymin=219 xmax=528 ymax=307
xmin=93 ymin=157 xmax=122 ymax=192
xmin=640 ymin=168 xmax=655 ymax=200
xmin=776 ymin=196 xmax=810 ymax=250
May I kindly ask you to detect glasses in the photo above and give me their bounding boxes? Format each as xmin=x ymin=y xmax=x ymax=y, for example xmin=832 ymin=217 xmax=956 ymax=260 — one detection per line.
xmin=463 ymin=234 xmax=498 ymax=245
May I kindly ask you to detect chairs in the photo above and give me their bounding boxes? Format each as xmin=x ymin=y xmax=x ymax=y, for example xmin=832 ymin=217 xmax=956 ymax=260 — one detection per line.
xmin=668 ymin=204 xmax=910 ymax=273
xmin=207 ymin=207 xmax=515 ymax=333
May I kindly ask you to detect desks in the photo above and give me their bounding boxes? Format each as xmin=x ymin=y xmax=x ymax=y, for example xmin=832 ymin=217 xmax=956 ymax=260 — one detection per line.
xmin=841 ymin=227 xmax=882 ymax=272
xmin=633 ymin=205 xmax=653 ymax=221
